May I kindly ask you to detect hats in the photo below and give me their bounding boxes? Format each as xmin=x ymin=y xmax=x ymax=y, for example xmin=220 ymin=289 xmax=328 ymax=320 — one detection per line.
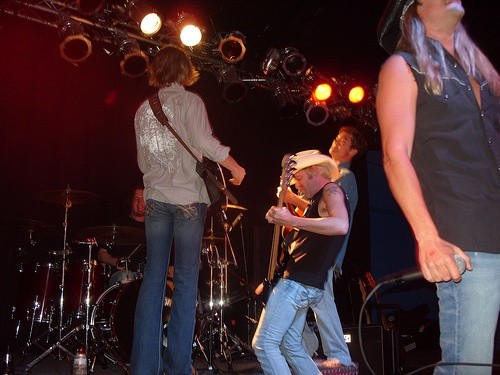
xmin=377 ymin=0 xmax=415 ymax=55
xmin=290 ymin=150 xmax=339 ymax=185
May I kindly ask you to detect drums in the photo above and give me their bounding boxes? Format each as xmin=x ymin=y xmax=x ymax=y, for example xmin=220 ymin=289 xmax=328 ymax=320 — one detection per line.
xmin=58 ymin=256 xmax=113 ymax=320
xmin=90 ymin=279 xmax=200 ymax=364
xmin=26 ymin=264 xmax=68 ymax=324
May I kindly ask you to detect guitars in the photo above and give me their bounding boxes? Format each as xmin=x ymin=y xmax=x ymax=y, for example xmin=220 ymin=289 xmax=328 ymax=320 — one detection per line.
xmin=199 ymin=157 xmax=228 ymax=212
xmin=262 ymin=153 xmax=297 ymax=305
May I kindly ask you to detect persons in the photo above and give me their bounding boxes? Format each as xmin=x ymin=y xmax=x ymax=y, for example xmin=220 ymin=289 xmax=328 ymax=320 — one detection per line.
xmin=308 ymin=125 xmax=366 ymax=368
xmin=376 ymin=0 xmax=500 ymax=375
xmin=132 ymin=44 xmax=246 ymax=375
xmin=96 ymin=181 xmax=174 ymax=367
xmin=252 ymin=148 xmax=351 ymax=375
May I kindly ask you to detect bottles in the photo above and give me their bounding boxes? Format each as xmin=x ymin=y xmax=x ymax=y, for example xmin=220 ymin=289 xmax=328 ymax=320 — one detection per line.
xmin=73 ymin=346 xmax=87 ymax=375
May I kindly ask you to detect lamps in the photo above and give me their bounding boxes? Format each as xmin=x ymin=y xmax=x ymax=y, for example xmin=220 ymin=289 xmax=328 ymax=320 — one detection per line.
xmin=123 ymin=0 xmax=163 ymax=37
xmin=114 ymin=32 xmax=149 ymax=77
xmin=218 ymin=31 xmax=366 ymax=126
xmin=57 ymin=11 xmax=92 ymax=63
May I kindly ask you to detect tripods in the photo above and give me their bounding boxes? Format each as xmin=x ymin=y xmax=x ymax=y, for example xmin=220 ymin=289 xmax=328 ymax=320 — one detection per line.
xmin=192 ymin=211 xmax=260 ymax=375
xmin=11 ymin=198 xmax=131 ymax=375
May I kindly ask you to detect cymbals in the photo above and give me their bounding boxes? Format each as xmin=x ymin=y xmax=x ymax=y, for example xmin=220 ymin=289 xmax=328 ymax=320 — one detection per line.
xmin=203 ymin=236 xmax=226 ymax=240
xmin=31 ymin=189 xmax=101 ymax=204
xmin=75 ymin=225 xmax=146 ymax=246
xmin=221 ymin=203 xmax=247 ymax=210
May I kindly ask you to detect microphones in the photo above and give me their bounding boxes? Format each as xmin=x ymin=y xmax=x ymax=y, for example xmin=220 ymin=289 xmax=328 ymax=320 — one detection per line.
xmin=382 ymin=254 xmax=467 ymax=284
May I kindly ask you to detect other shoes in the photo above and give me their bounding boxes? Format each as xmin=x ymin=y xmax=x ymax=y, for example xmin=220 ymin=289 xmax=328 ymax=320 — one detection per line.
xmin=323 ymin=359 xmax=341 ymax=368
